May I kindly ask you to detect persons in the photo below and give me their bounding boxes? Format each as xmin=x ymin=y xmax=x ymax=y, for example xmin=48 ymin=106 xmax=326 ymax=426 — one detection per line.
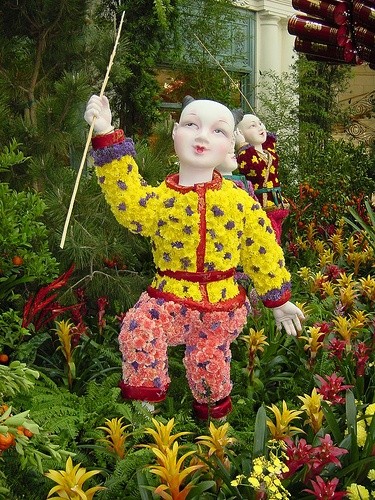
xmin=232 ymin=110 xmax=289 ymax=244
xmin=84 ymin=93 xmax=304 ymax=427
